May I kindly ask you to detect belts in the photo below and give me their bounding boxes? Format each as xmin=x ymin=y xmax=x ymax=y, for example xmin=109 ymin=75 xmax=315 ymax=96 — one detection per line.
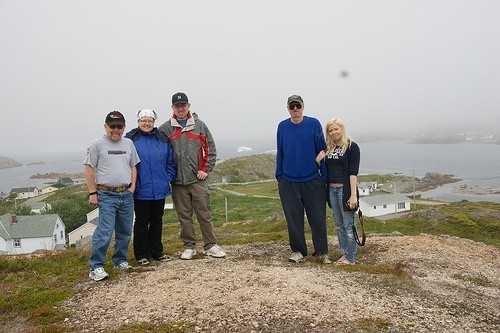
xmin=98 ymin=185 xmax=129 ymax=193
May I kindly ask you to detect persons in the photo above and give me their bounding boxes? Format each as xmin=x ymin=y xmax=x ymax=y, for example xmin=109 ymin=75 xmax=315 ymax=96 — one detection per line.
xmin=157 ymin=93 xmax=226 ymax=260
xmin=82 ymin=110 xmax=141 ymax=281
xmin=275 ymin=95 xmax=331 ymax=264
xmin=87 ymin=107 xmax=176 ymax=265
xmin=315 ymin=119 xmax=360 ymax=265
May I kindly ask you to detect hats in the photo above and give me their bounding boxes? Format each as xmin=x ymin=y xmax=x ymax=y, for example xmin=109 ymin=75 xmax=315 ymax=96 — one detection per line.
xmin=171 ymin=92 xmax=188 ymax=105
xmin=105 ymin=111 xmax=125 ymax=125
xmin=287 ymin=95 xmax=303 ymax=104
xmin=136 ymin=109 xmax=157 ymax=122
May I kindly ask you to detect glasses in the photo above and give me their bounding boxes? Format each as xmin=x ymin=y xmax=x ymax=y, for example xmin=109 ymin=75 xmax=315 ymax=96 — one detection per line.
xmin=289 ymin=104 xmax=302 ymax=110
xmin=109 ymin=124 xmax=123 ymax=129
xmin=174 ymin=104 xmax=186 ymax=108
xmin=140 ymin=119 xmax=153 ymax=123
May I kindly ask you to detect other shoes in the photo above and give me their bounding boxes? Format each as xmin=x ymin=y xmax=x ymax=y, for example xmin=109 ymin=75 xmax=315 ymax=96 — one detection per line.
xmin=337 ymin=256 xmax=355 ymax=266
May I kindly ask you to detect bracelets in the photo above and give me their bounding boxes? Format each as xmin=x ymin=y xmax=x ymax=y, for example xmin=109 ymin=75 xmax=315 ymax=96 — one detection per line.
xmin=89 ymin=191 xmax=97 ymax=195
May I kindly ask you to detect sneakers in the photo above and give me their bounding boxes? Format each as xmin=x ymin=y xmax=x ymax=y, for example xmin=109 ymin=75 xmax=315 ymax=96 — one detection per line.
xmin=137 ymin=258 xmax=150 ymax=266
xmin=289 ymin=251 xmax=305 ymax=264
xmin=181 ymin=249 xmax=197 ymax=259
xmin=313 ymin=255 xmax=332 ymax=264
xmin=202 ymin=245 xmax=226 ymax=258
xmin=88 ymin=267 xmax=109 ymax=282
xmin=159 ymin=255 xmax=172 ymax=262
xmin=114 ymin=262 xmax=133 ymax=272
